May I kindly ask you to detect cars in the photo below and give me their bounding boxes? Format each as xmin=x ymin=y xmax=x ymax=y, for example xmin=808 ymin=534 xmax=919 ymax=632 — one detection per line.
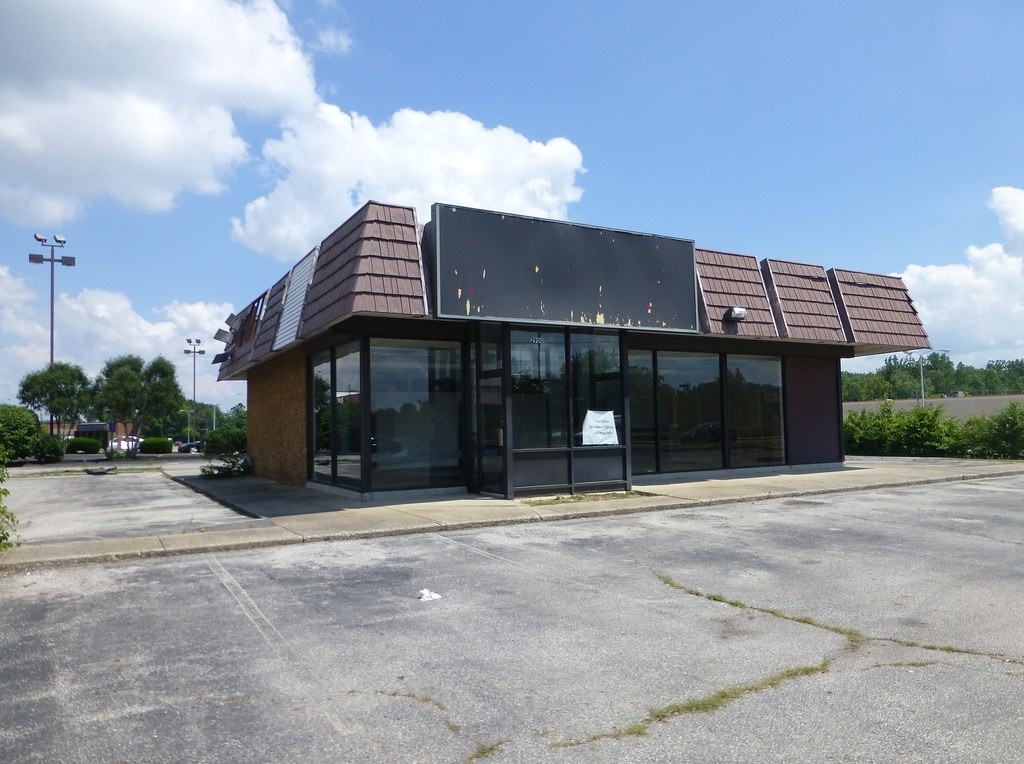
xmin=108 ymin=435 xmax=144 ymax=454
xmin=178 ymin=440 xmax=207 ymax=454
xmin=370 ymin=435 xmax=402 ymax=455
xmin=680 ymin=422 xmax=737 ymax=444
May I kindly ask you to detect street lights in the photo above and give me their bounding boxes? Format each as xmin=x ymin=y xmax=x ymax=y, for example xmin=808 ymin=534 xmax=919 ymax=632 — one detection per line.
xmin=29 ymin=232 xmax=76 ymax=434
xmin=183 ymin=339 xmax=205 ymax=442
xmin=903 ymin=348 xmax=951 ymax=408
